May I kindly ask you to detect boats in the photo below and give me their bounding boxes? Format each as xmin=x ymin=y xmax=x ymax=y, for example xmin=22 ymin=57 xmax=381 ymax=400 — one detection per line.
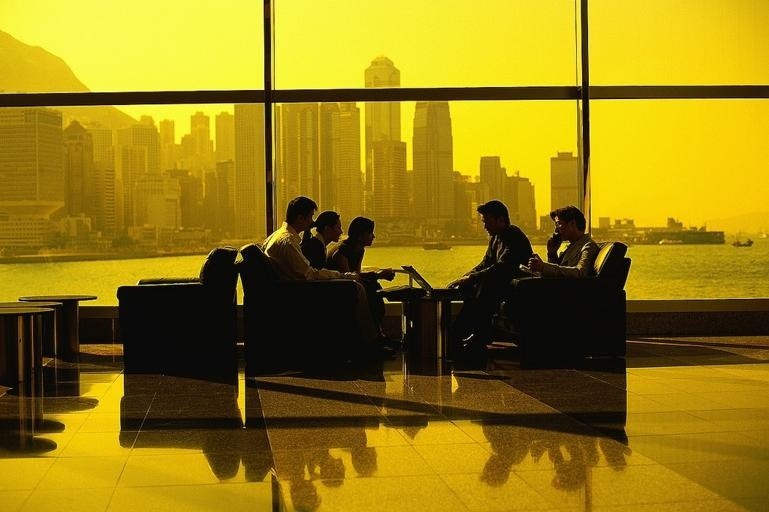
xmin=423 ymin=242 xmax=452 ymax=250
xmin=731 ymin=239 xmax=753 ymax=246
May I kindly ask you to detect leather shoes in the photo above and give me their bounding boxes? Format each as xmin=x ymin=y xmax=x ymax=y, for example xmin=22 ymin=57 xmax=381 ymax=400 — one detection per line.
xmin=455 ymin=333 xmax=478 ymax=352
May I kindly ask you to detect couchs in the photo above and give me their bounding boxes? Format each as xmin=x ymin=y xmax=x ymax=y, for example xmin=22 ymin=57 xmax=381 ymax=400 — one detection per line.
xmin=505 ymin=241 xmax=631 ymax=356
xmin=117 ymin=246 xmax=238 ymax=372
xmin=240 ymin=243 xmax=357 ymax=368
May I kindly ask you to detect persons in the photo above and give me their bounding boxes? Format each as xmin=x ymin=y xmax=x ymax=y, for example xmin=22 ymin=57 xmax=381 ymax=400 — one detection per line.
xmin=263 ymin=194 xmax=397 ymax=361
xmin=265 ymin=427 xmax=377 ymax=512
xmin=453 ymin=205 xmax=599 ymax=372
xmin=299 ymin=210 xmax=344 ymax=267
xmin=324 ymin=213 xmax=395 ymax=342
xmin=443 ymin=197 xmax=534 ymax=349
xmin=479 ymin=424 xmax=630 ymax=491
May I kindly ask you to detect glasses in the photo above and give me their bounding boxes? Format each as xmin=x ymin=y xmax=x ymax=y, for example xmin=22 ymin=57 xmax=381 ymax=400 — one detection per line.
xmin=553 ymin=221 xmax=571 ymax=229
